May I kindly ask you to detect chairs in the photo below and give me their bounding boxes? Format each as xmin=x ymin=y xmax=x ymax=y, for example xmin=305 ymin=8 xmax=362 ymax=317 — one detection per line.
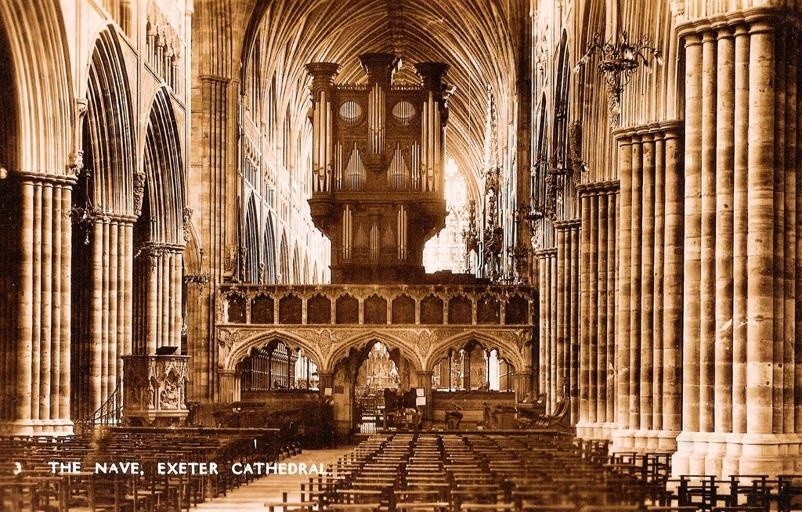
xmin=0 ymin=427 xmax=801 ymax=512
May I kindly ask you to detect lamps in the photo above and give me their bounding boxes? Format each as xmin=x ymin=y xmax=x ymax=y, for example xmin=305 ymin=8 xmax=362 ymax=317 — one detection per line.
xmin=513 ymin=199 xmax=547 ymax=243
xmin=462 ymin=198 xmax=480 ymax=269
xmin=482 ymin=166 xmax=502 ymax=228
xmin=65 ymin=167 xmax=107 ymax=246
xmin=480 ymin=228 xmax=531 ymax=284
xmin=531 ymin=140 xmax=590 ymax=197
xmin=184 ymin=251 xmax=214 ymax=298
xmin=574 ymin=30 xmax=665 ymax=129
